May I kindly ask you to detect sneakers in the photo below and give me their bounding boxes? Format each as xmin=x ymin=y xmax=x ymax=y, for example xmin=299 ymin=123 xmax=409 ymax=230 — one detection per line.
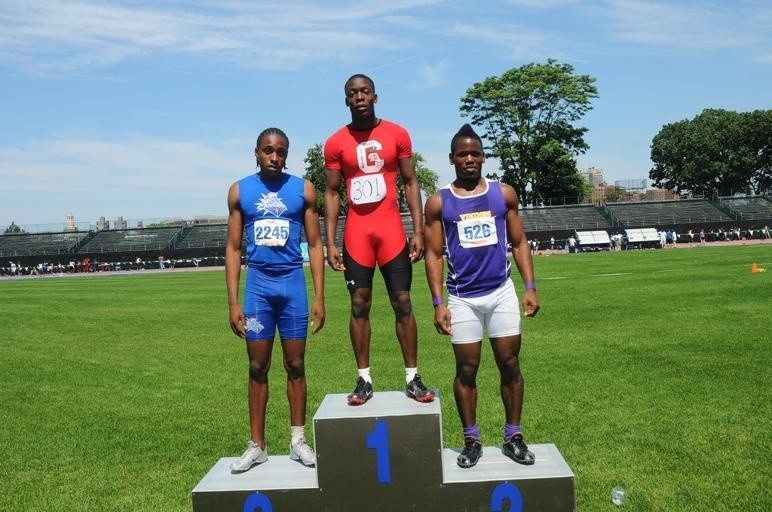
xmin=501 ymin=431 xmax=537 ymax=467
xmin=455 ymin=435 xmax=484 ymax=469
xmin=404 ymin=373 xmax=436 ymax=402
xmin=289 ymin=437 xmax=316 ymax=465
xmin=346 ymin=374 xmax=374 ymax=405
xmin=229 ymin=440 xmax=268 ymax=471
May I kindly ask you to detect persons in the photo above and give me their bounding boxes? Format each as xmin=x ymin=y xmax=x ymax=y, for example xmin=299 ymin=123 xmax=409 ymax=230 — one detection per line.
xmin=698 ymin=230 xmax=706 ymax=245
xmin=423 ymin=123 xmax=540 ymax=468
xmin=9 ymin=255 xmax=177 ymax=276
xmin=615 ymin=231 xmax=626 ymax=251
xmin=667 ymin=230 xmax=672 ymax=246
xmin=550 ymin=236 xmax=555 ymax=250
xmin=323 ymin=74 xmax=436 ymax=406
xmin=658 ymin=229 xmax=666 ymax=246
xmin=224 ymin=128 xmax=325 ymax=471
xmin=672 ymin=229 xmax=677 ymax=247
xmin=568 ymin=236 xmax=575 ymax=253
xmin=610 ymin=232 xmax=616 ymax=250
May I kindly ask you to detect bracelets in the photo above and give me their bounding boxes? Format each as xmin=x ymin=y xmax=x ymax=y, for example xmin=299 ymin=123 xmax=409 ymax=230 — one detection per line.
xmin=431 ymin=296 xmax=443 ymax=306
xmin=525 ymin=283 xmax=538 ymax=290
